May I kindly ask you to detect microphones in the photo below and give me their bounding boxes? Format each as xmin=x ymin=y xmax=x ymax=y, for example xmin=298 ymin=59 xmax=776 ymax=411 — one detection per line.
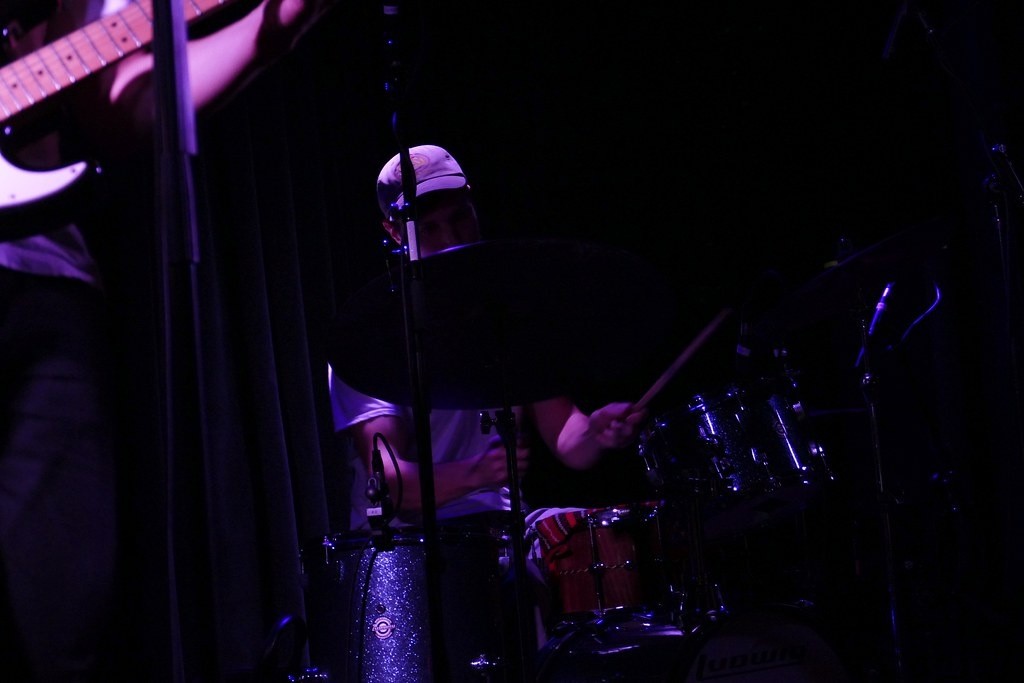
xmin=372 ymin=450 xmax=396 ymax=504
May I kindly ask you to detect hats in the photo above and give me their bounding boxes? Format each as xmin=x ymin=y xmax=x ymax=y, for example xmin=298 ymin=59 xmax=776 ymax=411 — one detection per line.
xmin=376 ymin=146 xmax=469 ymax=222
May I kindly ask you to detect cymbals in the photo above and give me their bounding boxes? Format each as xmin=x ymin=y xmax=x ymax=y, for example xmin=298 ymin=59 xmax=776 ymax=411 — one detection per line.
xmin=317 ymin=236 xmax=671 ymax=411
xmin=754 ymin=213 xmax=952 ymax=337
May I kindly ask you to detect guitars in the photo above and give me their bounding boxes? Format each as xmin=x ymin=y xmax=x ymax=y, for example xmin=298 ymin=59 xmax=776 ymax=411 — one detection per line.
xmin=0 ymin=1 xmax=270 ymax=217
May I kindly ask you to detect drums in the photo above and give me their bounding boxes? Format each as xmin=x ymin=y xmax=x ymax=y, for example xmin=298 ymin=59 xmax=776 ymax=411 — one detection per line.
xmin=311 ymin=508 xmax=512 ymax=683
xmin=521 ymin=498 xmax=708 ymax=612
xmin=517 ymin=600 xmax=863 ymax=682
xmin=634 ymin=381 xmax=832 ymax=542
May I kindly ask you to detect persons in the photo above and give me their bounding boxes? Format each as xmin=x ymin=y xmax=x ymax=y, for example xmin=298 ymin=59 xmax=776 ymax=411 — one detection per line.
xmin=0 ymin=0 xmax=330 ymax=683
xmin=327 ymin=145 xmax=644 ymax=683
